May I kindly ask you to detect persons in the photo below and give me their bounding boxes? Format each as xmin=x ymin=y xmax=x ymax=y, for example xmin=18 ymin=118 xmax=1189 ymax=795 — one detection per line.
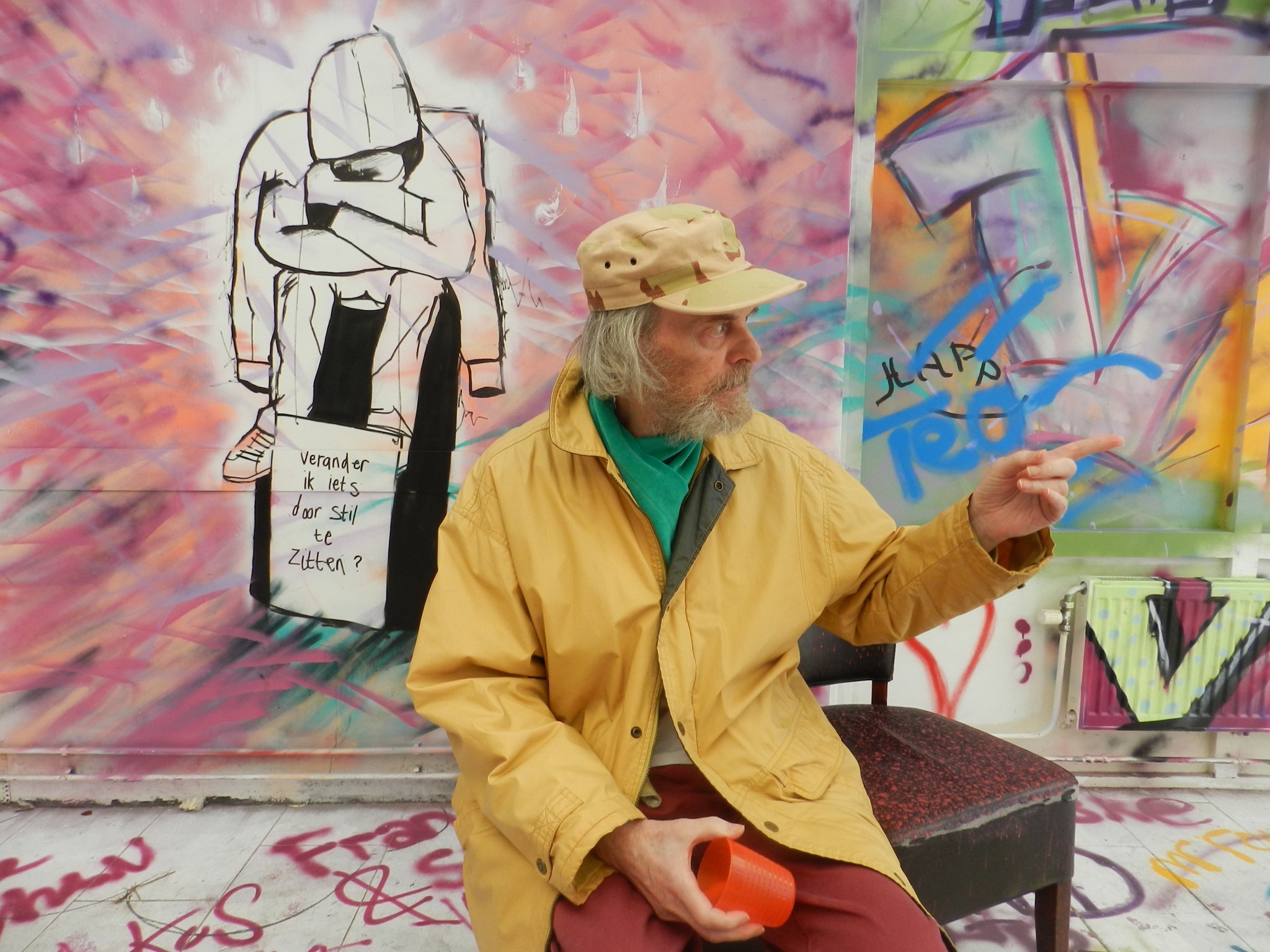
xmin=403 ymin=205 xmax=1122 ymax=952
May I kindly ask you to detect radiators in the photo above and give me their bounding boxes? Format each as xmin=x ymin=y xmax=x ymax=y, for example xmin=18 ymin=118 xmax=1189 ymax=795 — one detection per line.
xmin=1037 ymin=576 xmax=1270 ymax=736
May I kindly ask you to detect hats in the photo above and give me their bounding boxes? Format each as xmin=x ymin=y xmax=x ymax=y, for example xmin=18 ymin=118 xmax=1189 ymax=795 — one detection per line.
xmin=576 ymin=203 xmax=808 ymax=316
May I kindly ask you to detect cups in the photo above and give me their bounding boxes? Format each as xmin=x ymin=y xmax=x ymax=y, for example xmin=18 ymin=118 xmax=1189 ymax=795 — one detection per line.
xmin=696 ymin=837 xmax=796 ymax=928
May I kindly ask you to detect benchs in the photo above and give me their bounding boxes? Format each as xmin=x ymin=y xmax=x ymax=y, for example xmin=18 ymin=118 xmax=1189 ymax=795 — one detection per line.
xmin=492 ymin=579 xmax=1089 ymax=952
